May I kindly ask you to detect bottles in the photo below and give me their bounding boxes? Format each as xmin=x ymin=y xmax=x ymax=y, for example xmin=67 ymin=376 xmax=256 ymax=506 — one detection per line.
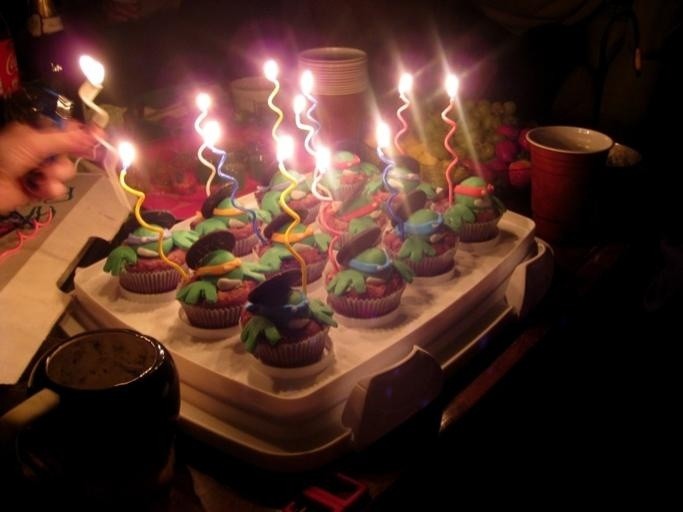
xmin=21 ymin=327 xmax=180 ymax=503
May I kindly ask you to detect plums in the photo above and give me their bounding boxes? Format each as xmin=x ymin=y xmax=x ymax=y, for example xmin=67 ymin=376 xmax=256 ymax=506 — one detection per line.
xmin=495 ymin=123 xmax=533 ymax=162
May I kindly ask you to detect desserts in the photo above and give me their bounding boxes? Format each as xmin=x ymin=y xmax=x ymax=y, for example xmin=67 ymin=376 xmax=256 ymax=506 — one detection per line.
xmin=104 ymin=138 xmax=508 ymax=367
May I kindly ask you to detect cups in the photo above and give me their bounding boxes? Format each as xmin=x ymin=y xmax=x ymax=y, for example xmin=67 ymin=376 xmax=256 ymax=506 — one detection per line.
xmin=299 ymin=45 xmax=369 ymax=155
xmin=524 ymin=126 xmax=614 ymax=247
xmin=231 ymin=75 xmax=293 ymax=119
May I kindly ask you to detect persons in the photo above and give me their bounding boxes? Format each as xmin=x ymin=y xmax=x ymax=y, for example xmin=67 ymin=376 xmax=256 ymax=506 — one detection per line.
xmin=1 ymin=111 xmax=103 ymax=218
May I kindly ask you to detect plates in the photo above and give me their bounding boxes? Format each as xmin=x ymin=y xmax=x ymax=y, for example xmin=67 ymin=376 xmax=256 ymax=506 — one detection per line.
xmin=132 ymin=129 xmax=291 ymax=222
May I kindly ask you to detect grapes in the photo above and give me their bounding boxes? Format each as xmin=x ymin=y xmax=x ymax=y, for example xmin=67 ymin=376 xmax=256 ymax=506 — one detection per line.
xmin=418 ymin=99 xmax=517 ymax=161
